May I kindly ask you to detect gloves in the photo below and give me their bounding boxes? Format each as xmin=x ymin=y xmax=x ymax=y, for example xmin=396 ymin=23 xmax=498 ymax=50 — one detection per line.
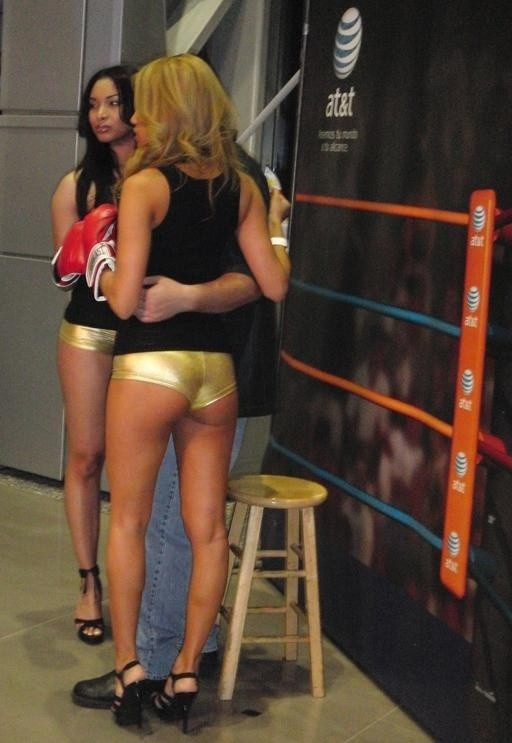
xmin=82 ymin=203 xmax=119 ymax=303
xmin=50 ymin=219 xmax=86 ymax=289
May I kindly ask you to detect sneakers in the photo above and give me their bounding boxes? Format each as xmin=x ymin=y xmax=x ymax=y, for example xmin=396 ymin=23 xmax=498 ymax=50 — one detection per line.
xmin=71 ymin=667 xmax=169 ymax=709
xmin=177 ymin=649 xmax=221 ymax=678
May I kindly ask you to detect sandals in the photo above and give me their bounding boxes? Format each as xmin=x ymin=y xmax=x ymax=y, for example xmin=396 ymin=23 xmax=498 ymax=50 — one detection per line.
xmin=74 ymin=565 xmax=105 ymax=645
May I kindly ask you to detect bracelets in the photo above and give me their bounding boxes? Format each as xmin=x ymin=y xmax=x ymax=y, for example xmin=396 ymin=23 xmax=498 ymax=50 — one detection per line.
xmin=267 ymin=235 xmax=290 ymax=250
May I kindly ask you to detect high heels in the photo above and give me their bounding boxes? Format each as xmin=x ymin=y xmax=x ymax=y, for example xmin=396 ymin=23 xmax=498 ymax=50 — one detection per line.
xmin=148 ymin=668 xmax=201 ymax=735
xmin=109 ymin=659 xmax=149 ymax=729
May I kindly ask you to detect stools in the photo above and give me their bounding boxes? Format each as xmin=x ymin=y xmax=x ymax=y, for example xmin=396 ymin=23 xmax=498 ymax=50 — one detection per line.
xmin=211 ymin=475 xmax=329 ymax=700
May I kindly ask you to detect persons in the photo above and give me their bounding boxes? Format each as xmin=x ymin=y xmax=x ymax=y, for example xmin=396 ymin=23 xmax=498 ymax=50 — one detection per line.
xmin=79 ymin=54 xmax=291 ymax=733
xmin=49 ymin=61 xmax=139 ymax=646
xmin=71 ymin=52 xmax=269 ymax=712
